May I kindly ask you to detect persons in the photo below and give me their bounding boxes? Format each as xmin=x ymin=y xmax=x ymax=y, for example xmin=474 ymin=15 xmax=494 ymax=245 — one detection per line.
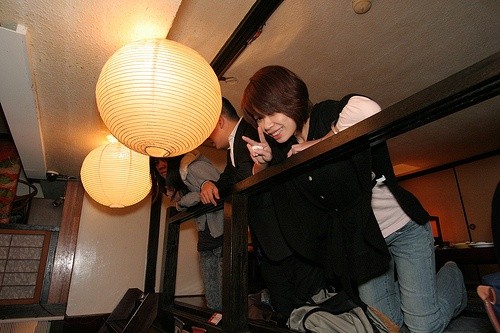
xmin=240 ymin=65 xmax=468 ymax=333
xmin=199 ymin=97 xmax=333 ymax=326
xmin=149 ymin=150 xmax=224 ymax=312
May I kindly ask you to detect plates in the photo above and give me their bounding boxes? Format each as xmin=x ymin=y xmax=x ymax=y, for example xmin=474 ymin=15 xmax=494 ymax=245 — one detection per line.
xmin=468 ymin=243 xmax=494 ymax=247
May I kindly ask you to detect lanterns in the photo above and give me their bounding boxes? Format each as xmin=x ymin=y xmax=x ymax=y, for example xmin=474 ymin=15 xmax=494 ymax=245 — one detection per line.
xmin=95 ymin=37 xmax=222 ymax=157
xmin=80 ymin=142 xmax=159 ymax=208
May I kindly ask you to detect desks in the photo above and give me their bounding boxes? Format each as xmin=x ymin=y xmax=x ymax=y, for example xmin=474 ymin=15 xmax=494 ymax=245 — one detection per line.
xmin=436 ymin=241 xmax=499 ymax=291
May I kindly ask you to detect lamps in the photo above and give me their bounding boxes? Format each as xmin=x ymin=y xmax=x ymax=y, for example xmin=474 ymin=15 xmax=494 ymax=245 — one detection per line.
xmin=80 ymin=142 xmax=153 ymax=208
xmin=95 ymin=38 xmax=221 ymax=157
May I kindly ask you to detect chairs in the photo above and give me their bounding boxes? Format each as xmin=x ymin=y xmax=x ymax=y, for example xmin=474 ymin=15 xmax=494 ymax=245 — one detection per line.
xmin=10 ymin=180 xmax=38 ymax=224
